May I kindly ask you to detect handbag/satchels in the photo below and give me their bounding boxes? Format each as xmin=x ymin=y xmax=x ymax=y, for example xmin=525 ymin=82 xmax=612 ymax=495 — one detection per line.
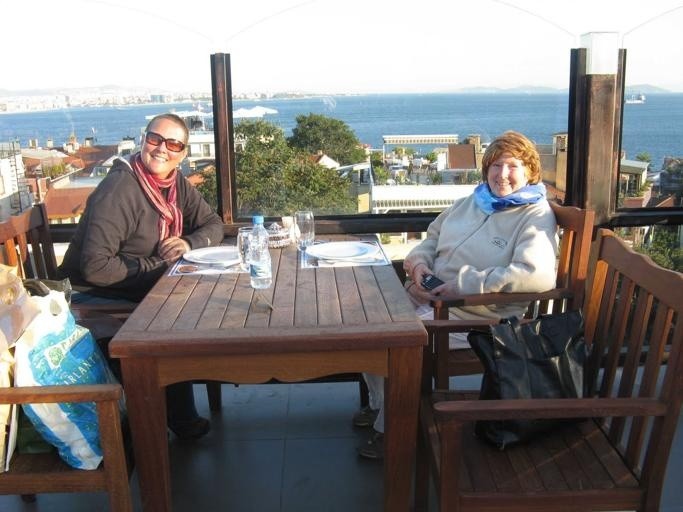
xmin=14 ymin=290 xmax=127 ymax=469
xmin=467 ymin=308 xmax=588 ymax=451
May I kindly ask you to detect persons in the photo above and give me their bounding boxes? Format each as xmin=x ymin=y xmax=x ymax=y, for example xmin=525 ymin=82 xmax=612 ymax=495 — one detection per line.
xmin=50 ymin=114 xmax=224 ymax=439
xmin=353 ymin=130 xmax=559 ymax=461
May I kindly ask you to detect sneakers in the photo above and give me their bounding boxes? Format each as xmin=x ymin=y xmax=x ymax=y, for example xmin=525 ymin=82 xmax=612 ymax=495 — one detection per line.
xmin=353 ymin=406 xmax=379 ymax=426
xmin=168 ymin=415 xmax=209 ymax=438
xmin=360 ymin=432 xmax=384 ymax=458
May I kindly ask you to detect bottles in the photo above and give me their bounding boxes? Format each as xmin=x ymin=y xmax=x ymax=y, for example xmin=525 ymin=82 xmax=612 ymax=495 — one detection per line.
xmin=248 ymin=215 xmax=273 ymax=291
xmin=268 ymin=214 xmax=300 ymax=242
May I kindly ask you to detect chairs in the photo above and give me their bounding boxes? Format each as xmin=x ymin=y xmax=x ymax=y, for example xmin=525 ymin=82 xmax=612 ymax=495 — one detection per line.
xmin=414 ymin=225 xmax=681 ymax=510
xmin=430 ymin=199 xmax=595 ymax=375
xmin=0 ymin=386 xmax=139 ymax=511
xmin=0 ymin=206 xmax=140 ymax=320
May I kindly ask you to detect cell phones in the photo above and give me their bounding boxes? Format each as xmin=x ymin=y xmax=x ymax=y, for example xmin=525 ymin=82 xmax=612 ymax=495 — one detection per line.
xmin=421 ymin=274 xmax=444 ymax=291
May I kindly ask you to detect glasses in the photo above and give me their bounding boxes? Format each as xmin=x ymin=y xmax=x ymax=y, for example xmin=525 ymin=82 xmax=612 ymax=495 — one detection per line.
xmin=145 ymin=132 xmax=184 ymax=152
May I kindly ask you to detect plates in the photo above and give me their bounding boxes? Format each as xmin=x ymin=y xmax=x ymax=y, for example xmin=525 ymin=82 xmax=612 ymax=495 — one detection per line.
xmin=182 ymin=245 xmax=241 ymax=268
xmin=305 ymin=240 xmax=380 ymax=259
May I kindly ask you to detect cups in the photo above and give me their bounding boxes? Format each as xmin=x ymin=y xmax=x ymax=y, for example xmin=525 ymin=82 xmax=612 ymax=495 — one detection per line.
xmin=292 ymin=209 xmax=316 ymax=251
xmin=235 ymin=224 xmax=255 ymax=272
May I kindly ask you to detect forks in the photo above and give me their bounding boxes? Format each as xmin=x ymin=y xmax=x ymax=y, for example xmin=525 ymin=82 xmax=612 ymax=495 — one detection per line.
xmin=322 ymin=256 xmax=382 ymax=265
xmin=195 ymin=263 xmax=240 ymax=269
